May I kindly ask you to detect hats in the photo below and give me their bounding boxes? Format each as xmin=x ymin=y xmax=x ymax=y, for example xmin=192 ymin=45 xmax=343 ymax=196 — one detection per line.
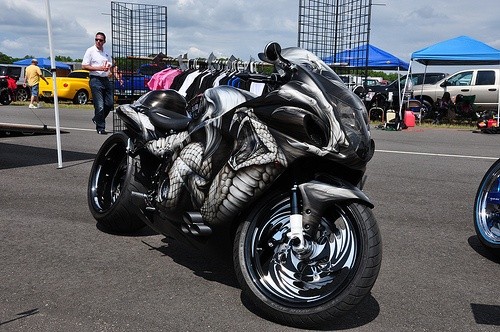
xmin=31 ymin=59 xmax=38 ymax=63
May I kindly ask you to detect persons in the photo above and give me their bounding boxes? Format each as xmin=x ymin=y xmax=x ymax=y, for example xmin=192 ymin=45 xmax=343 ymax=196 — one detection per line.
xmin=439 ymin=92 xmax=477 ymax=124
xmin=22 ymin=58 xmax=49 ymax=108
xmin=82 ymin=32 xmax=114 ymax=134
xmin=371 ymin=93 xmax=388 ymax=119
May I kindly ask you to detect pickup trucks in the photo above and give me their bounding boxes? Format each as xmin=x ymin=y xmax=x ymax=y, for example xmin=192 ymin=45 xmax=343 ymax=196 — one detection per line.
xmin=360 ymin=71 xmax=451 ymax=112
xmin=114 ymin=63 xmax=172 ymax=105
xmin=402 ymin=69 xmax=500 ymax=120
xmin=38 ymin=70 xmax=119 ymax=105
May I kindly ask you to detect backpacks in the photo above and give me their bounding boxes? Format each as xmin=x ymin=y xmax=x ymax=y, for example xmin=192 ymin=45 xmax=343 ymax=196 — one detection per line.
xmin=7 ymin=77 xmax=17 ymax=90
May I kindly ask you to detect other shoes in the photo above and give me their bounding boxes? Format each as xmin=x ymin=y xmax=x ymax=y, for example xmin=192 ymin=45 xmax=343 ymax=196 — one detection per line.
xmin=97 ymin=129 xmax=107 ymax=134
xmin=37 ymin=103 xmax=43 ymax=109
xmin=92 ymin=118 xmax=96 ymax=124
xmin=28 ymin=104 xmax=38 ymax=108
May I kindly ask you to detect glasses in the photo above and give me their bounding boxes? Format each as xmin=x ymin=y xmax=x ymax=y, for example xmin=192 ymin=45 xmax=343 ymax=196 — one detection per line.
xmin=95 ymin=38 xmax=105 ymax=42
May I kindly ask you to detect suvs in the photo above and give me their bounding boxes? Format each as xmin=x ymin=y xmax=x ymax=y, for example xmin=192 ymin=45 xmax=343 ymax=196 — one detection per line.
xmin=0 ymin=63 xmax=53 ymax=102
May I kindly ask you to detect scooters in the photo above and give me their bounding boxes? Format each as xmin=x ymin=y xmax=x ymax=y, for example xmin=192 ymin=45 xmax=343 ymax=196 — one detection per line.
xmin=0 ymin=70 xmax=20 ymax=106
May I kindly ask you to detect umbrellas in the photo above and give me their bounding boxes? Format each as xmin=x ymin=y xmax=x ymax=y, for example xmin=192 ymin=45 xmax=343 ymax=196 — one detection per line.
xmin=13 ymin=58 xmax=71 ymax=77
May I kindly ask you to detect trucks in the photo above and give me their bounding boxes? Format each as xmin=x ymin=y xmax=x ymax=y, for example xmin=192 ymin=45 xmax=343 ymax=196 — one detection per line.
xmin=338 ymin=74 xmax=384 ymax=92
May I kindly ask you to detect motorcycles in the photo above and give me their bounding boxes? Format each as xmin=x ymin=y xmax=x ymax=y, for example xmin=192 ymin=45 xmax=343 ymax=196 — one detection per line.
xmin=87 ymin=39 xmax=384 ymax=325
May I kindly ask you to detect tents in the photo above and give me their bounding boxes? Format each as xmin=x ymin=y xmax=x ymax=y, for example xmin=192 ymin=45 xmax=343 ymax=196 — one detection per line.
xmin=319 ymin=43 xmax=412 ymax=130
xmin=397 ymin=36 xmax=500 ymax=132
